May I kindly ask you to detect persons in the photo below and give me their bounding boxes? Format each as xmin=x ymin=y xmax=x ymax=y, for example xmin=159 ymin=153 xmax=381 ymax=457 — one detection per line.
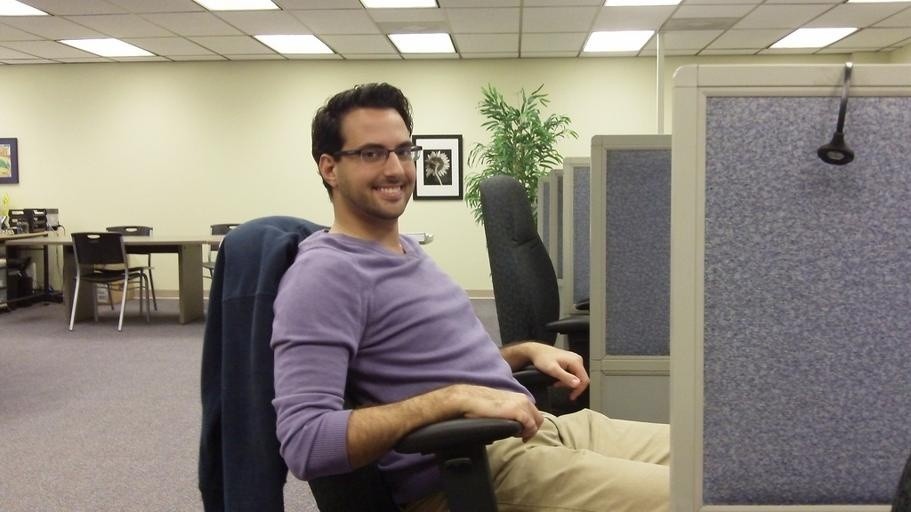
xmin=271 ymin=82 xmax=671 ymax=512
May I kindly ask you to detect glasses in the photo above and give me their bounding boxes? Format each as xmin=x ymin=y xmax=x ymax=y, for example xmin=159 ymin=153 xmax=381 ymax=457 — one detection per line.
xmin=332 ymin=144 xmax=422 ymax=167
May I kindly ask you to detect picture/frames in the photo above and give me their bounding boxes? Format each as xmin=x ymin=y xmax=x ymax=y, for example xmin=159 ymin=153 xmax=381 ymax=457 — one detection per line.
xmin=411 ymin=135 xmax=462 ymax=200
xmin=0 ymin=138 xmax=19 ymax=184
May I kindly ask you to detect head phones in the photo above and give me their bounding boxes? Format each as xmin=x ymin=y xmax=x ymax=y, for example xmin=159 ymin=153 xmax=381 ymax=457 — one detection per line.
xmin=818 ymin=62 xmax=855 ymax=166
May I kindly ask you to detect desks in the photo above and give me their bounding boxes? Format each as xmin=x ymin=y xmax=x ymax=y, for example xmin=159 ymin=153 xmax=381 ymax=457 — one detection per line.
xmin=5 ymin=232 xmax=224 ymax=324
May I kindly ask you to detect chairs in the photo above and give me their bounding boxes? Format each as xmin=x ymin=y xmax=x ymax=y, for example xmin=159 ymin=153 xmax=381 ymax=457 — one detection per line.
xmin=69 ymin=225 xmax=240 ymax=331
xmin=475 ymin=178 xmax=588 ymax=417
xmin=244 ymin=217 xmax=570 ymax=512
